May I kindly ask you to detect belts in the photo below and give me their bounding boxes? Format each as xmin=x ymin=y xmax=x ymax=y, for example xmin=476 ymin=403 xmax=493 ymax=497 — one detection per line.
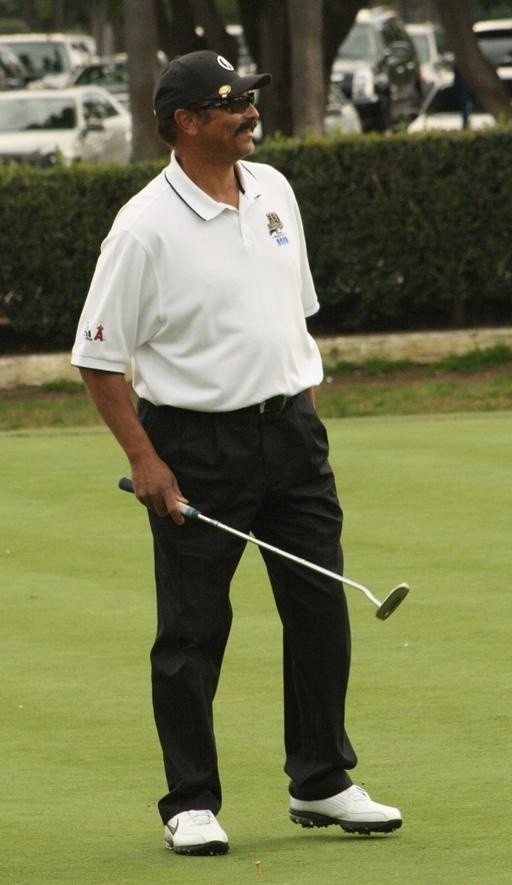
xmin=251 ymin=394 xmax=294 ymax=414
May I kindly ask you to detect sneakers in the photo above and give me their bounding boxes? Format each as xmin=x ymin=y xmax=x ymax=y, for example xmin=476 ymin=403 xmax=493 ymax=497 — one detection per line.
xmin=162 ymin=808 xmax=230 ymax=857
xmin=289 ymin=782 xmax=403 ymax=833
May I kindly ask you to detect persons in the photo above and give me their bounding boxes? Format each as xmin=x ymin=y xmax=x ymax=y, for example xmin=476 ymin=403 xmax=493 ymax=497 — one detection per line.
xmin=67 ymin=48 xmax=407 ymax=856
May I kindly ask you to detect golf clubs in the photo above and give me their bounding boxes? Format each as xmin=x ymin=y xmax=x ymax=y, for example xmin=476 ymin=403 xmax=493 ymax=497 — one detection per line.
xmin=118 ymin=477 xmax=409 ymax=619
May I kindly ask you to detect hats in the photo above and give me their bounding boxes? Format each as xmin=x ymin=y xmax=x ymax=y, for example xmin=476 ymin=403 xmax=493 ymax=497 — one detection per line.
xmin=154 ymin=49 xmax=272 ymax=119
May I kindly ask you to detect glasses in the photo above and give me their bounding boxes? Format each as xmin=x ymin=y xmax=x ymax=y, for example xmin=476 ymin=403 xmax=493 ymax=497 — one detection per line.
xmin=192 ymin=91 xmax=257 ymax=114
